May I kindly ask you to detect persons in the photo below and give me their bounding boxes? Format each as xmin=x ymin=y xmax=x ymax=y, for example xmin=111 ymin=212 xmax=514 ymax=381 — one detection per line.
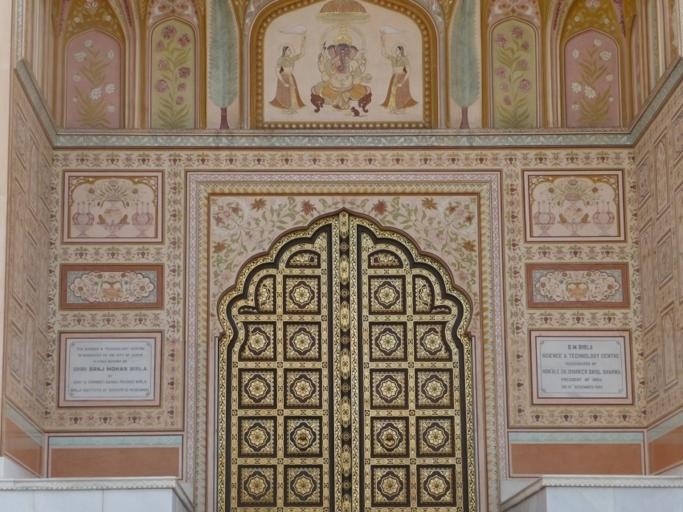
xmin=310 ymin=43 xmax=371 ymax=110
xmin=269 ymin=39 xmax=304 ymax=114
xmin=379 ymin=35 xmax=417 ymax=114
